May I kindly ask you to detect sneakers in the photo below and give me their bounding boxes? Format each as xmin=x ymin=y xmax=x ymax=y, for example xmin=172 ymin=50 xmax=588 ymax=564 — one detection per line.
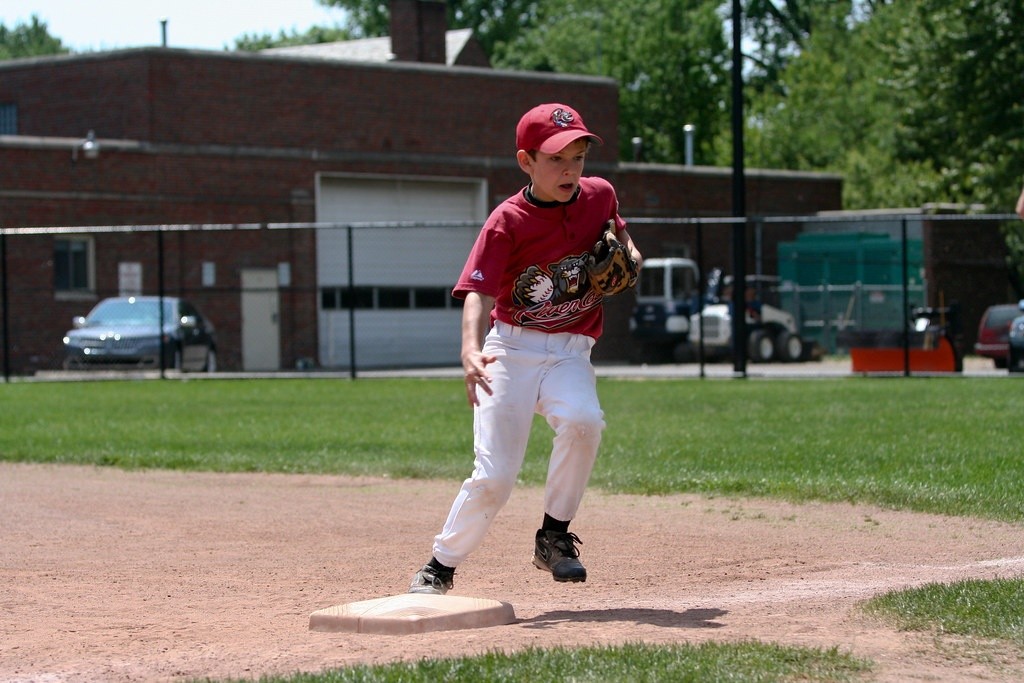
xmin=407 ymin=563 xmax=455 ymax=595
xmin=531 ymin=528 xmax=587 ymax=582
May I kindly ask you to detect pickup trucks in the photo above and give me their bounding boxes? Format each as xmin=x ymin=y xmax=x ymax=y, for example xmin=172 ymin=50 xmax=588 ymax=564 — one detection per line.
xmin=628 ymin=257 xmax=768 ymax=343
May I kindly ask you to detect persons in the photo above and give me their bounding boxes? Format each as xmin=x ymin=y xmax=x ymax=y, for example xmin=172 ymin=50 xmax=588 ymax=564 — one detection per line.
xmin=406 ymin=103 xmax=643 ymax=595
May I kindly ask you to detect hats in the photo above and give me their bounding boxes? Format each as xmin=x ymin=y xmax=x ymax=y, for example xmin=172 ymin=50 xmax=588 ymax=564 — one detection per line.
xmin=516 ymin=103 xmax=603 ymax=155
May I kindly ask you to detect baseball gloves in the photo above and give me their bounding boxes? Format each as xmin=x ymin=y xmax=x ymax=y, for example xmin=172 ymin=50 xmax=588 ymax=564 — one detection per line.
xmin=585 ymin=216 xmax=640 ymax=300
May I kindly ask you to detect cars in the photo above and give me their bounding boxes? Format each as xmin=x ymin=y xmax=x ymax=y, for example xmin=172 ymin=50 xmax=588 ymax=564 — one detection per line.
xmin=974 ymin=300 xmax=1024 ymax=374
xmin=59 ymin=294 xmax=218 ymax=374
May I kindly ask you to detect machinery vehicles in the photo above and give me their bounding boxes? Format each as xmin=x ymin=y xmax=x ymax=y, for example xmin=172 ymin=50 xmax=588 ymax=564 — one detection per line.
xmin=669 ymin=273 xmax=802 ymax=364
